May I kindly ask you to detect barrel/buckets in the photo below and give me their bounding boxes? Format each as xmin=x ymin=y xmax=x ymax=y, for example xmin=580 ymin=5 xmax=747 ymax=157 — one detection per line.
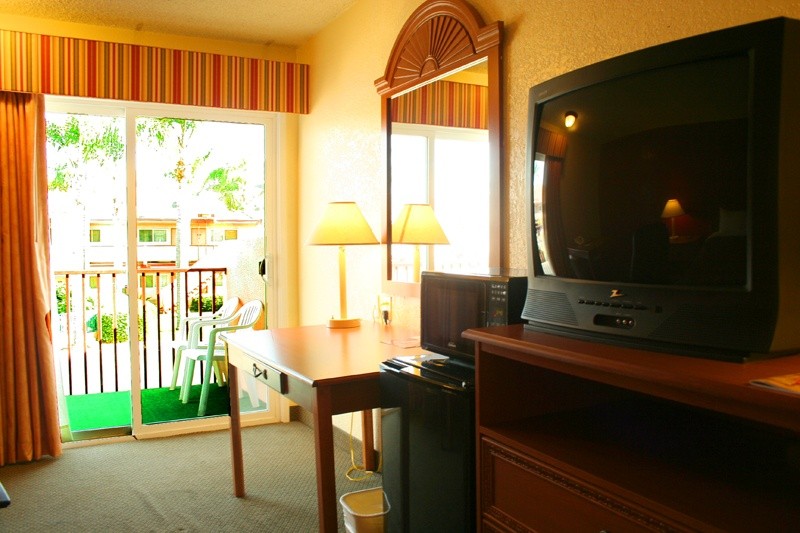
xmin=340 ymin=487 xmax=384 ymax=533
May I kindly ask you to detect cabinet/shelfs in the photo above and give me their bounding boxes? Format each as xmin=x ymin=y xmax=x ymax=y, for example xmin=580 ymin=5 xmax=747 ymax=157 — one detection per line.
xmin=460 ymin=324 xmax=800 ymax=533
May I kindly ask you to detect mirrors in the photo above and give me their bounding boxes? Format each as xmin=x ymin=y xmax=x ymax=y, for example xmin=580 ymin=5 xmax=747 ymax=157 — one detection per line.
xmin=374 ymin=0 xmax=507 ymax=282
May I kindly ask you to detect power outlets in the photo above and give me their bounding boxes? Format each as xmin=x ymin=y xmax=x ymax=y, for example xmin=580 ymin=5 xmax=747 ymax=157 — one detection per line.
xmin=377 ymin=294 xmax=392 ymax=321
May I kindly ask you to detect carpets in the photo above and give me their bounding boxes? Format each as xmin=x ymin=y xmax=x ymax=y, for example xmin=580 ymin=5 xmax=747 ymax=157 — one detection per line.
xmin=65 ymin=382 xmax=267 ymax=432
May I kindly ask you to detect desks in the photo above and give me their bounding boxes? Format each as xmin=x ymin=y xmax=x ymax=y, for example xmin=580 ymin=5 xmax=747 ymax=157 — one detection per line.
xmin=218 ymin=325 xmax=470 ymax=533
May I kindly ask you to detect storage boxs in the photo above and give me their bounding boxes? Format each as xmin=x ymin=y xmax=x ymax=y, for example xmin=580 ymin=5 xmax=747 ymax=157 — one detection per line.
xmin=340 ymin=487 xmax=391 ymax=533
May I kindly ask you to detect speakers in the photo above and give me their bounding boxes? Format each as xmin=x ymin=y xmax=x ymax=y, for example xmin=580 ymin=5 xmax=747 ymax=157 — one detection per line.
xmin=378 ymin=358 xmax=476 ymax=532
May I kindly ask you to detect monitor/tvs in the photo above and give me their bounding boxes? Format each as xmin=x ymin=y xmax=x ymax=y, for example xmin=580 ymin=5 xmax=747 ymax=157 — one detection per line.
xmin=520 ymin=16 xmax=800 ymax=365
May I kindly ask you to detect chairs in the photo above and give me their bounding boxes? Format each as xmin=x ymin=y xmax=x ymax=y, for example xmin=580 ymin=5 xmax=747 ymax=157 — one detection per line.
xmin=169 ymin=298 xmax=263 ymax=416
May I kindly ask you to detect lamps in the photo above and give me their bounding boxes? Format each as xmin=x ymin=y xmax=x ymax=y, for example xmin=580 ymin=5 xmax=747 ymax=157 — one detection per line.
xmin=382 ymin=203 xmax=451 ymax=282
xmin=661 ymin=199 xmax=684 ymax=239
xmin=307 ymin=202 xmax=380 ymax=328
xmin=565 ymin=111 xmax=577 ymax=128
